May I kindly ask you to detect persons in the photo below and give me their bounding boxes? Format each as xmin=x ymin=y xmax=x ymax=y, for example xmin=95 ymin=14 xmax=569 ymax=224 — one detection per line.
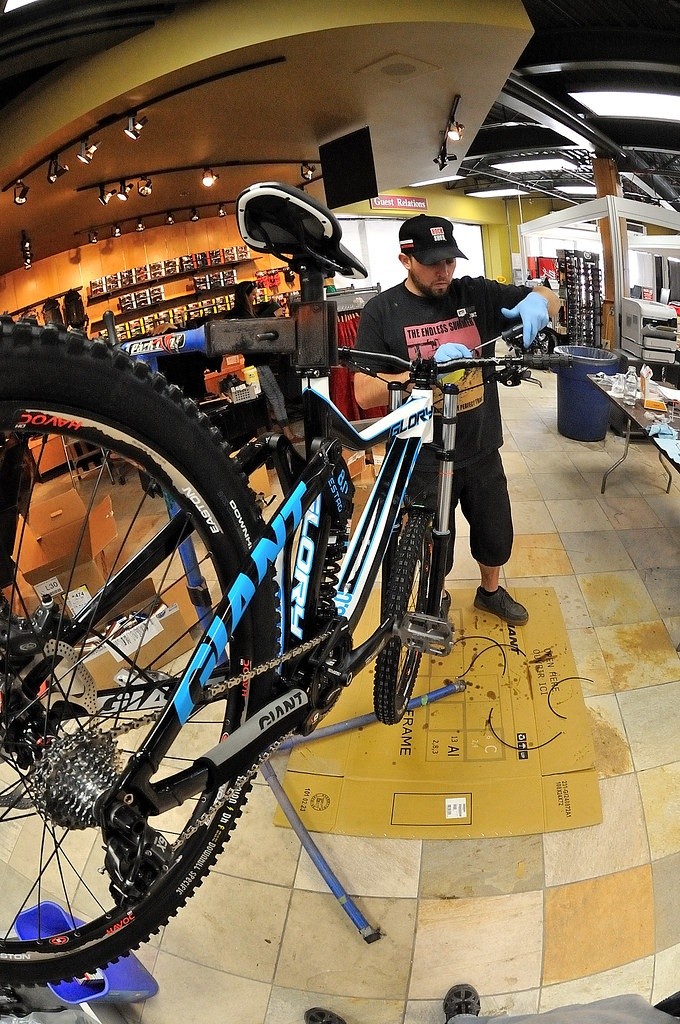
xmin=354 ymin=214 xmax=562 ymax=629
xmin=224 ymin=281 xmax=305 ymax=444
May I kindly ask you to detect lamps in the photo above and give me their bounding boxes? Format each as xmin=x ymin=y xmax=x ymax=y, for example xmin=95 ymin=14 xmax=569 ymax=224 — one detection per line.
xmin=13 ymin=111 xmax=316 ymax=270
xmin=433 ymin=87 xmax=680 ymax=197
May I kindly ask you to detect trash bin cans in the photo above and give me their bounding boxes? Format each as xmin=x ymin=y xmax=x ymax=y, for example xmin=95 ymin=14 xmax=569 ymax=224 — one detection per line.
xmin=553 ymin=345 xmax=618 ymax=442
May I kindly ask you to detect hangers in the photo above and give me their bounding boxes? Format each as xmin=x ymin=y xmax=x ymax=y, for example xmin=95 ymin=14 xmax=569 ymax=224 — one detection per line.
xmin=337 ymin=310 xmax=360 ymax=322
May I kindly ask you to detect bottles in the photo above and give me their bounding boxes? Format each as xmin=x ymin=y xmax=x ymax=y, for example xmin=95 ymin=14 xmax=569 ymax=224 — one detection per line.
xmin=622 ymin=366 xmax=638 ymax=406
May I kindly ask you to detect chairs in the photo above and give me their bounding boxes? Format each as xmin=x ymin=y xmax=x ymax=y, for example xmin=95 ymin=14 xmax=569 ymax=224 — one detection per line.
xmin=206 ymin=397 xmax=270 ymax=451
xmin=61 ymin=435 xmax=115 ymax=490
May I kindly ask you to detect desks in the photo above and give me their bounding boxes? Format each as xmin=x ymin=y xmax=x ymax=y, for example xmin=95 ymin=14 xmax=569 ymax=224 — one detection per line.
xmin=587 ymin=373 xmax=680 ymax=494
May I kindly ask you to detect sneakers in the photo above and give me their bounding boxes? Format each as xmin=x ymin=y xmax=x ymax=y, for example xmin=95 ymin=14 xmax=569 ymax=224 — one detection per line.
xmin=303 ymin=1007 xmax=346 ymax=1024
xmin=439 ymin=589 xmax=452 ymax=619
xmin=444 ymin=985 xmax=480 ymax=1023
xmin=474 ymin=586 xmax=528 ymax=626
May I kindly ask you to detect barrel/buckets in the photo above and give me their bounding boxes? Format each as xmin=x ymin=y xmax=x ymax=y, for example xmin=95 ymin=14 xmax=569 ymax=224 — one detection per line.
xmin=13 ymin=900 xmax=160 ymax=1005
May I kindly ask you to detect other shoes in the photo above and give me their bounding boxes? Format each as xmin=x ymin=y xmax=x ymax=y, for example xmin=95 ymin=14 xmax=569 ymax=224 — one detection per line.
xmin=289 ymin=435 xmax=305 ymax=444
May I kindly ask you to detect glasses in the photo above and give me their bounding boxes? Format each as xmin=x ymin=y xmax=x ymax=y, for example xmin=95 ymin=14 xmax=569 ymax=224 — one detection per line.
xmin=246 ymin=281 xmax=257 ymax=292
xmin=556 ymin=259 xmax=603 ymax=347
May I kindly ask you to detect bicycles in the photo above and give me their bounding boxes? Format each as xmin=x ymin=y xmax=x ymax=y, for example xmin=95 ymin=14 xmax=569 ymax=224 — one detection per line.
xmin=0 ymin=182 xmax=568 ymax=989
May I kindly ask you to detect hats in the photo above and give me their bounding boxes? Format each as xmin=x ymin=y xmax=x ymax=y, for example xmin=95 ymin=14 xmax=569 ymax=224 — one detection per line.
xmin=398 ymin=213 xmax=469 ymax=265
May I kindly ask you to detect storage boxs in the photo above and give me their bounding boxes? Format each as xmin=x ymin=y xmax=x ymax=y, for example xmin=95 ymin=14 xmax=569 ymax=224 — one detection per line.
xmin=0 ymin=442 xmax=387 ymax=689
xmin=231 ymin=384 xmax=256 ymax=405
xmin=90 ymin=245 xmax=268 ymax=343
xmin=241 ymin=365 xmax=261 ymax=395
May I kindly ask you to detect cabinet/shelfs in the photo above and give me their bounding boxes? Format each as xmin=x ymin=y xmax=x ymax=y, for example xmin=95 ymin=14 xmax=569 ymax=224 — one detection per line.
xmin=88 ymin=257 xmax=264 ymax=325
xmin=608 ymin=349 xmax=680 ymax=443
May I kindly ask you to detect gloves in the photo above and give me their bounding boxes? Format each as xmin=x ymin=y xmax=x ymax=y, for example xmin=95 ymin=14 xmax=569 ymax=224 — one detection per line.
xmin=501 ymin=293 xmax=548 ymax=348
xmin=431 ymin=345 xmax=471 ymax=381
